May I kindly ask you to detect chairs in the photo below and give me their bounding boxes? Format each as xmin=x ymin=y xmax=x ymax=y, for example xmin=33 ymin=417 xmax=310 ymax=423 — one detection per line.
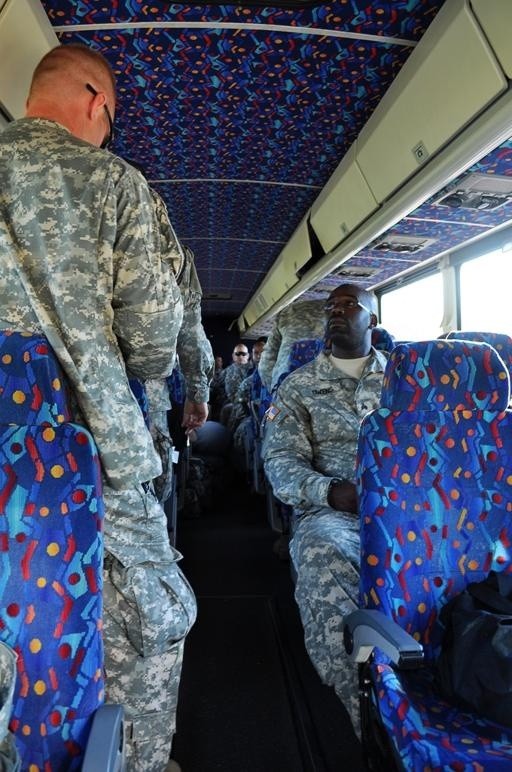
xmin=245 ymin=334 xmax=512 ymax=769
xmin=0 ymin=331 xmax=190 ymax=771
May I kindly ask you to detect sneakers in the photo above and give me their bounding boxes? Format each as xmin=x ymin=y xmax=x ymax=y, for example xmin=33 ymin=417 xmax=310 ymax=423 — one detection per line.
xmin=270 ymin=533 xmax=293 ymax=561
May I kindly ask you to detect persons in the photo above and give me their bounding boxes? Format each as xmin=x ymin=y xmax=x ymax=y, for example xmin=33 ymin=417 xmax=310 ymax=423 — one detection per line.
xmin=255 ymin=299 xmax=333 ymax=393
xmin=0 ymin=40 xmax=203 ymax=771
xmin=132 ymin=240 xmax=217 ymax=508
xmin=259 ymin=283 xmax=392 ymax=743
xmin=168 ymin=334 xmax=279 ymax=512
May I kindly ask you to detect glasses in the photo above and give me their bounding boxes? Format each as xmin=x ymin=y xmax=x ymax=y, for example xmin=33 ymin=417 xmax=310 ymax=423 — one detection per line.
xmin=84 ymin=82 xmax=118 ymax=150
xmin=321 ymin=298 xmax=375 ymax=316
